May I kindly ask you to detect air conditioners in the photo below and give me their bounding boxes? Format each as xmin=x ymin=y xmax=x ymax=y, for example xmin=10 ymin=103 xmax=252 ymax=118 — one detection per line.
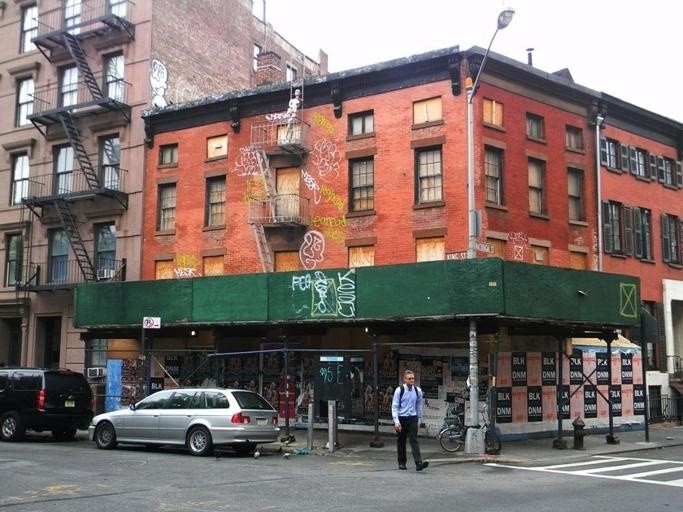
xmin=97 ymin=269 xmax=115 ymax=279
xmin=87 ymin=367 xmax=106 ymax=379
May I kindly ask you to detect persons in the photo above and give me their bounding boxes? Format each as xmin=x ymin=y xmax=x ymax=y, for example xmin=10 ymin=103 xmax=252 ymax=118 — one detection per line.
xmin=284 ymin=89 xmax=303 ymax=140
xmin=391 ymin=368 xmax=429 ymax=472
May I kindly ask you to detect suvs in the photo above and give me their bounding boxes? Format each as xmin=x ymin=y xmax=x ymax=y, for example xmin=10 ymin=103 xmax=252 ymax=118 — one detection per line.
xmin=0 ymin=365 xmax=95 ymax=441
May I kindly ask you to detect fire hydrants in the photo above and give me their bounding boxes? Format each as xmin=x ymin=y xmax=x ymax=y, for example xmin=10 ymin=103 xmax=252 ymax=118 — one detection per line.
xmin=569 ymin=415 xmax=587 ymax=451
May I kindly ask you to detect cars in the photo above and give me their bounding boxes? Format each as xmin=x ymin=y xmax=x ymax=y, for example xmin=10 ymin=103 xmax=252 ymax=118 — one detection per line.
xmin=86 ymin=387 xmax=280 ymax=459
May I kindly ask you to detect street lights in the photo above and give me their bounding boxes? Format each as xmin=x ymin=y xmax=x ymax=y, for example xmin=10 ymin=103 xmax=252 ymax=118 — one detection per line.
xmin=463 ymin=4 xmax=516 ymax=455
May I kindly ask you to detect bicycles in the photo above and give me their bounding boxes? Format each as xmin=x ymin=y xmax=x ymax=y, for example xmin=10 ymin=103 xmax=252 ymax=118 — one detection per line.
xmin=437 ymin=407 xmax=502 ymax=455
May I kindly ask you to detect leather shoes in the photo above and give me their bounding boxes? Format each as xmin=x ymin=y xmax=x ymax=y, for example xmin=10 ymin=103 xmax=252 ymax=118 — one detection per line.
xmin=399 ymin=463 xmax=406 ymax=470
xmin=416 ymin=462 xmax=427 ymax=471
